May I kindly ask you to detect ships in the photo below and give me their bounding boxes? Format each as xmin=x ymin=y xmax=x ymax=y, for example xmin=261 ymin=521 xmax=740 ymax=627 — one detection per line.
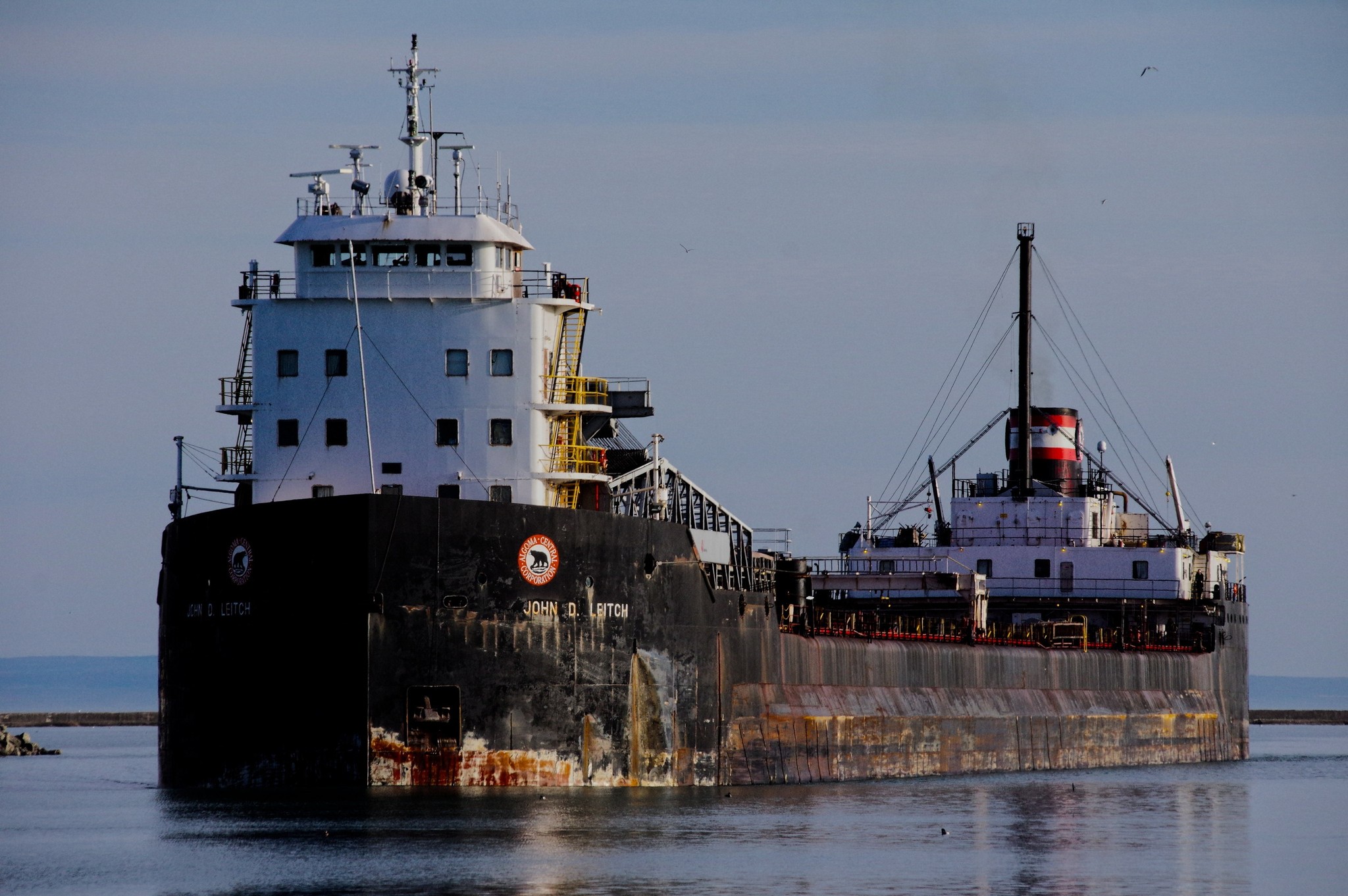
xmin=154 ymin=33 xmax=1249 ymax=808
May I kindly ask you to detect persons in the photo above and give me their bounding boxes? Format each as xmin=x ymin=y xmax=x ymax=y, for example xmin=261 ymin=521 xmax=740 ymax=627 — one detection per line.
xmin=271 ymin=273 xmax=280 ymax=298
xmin=1129 ymin=629 xmax=1141 ymax=645
xmin=1234 ymin=584 xmax=1238 ymax=601
xmin=1196 ymin=569 xmax=1204 ymax=598
xmin=849 ymin=613 xmax=856 ymax=632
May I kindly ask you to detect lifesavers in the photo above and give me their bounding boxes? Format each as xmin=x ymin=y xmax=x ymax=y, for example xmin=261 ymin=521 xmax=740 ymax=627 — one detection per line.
xmin=1234 ymin=584 xmax=1237 ymax=595
xmin=600 ymin=450 xmax=607 ymax=467
